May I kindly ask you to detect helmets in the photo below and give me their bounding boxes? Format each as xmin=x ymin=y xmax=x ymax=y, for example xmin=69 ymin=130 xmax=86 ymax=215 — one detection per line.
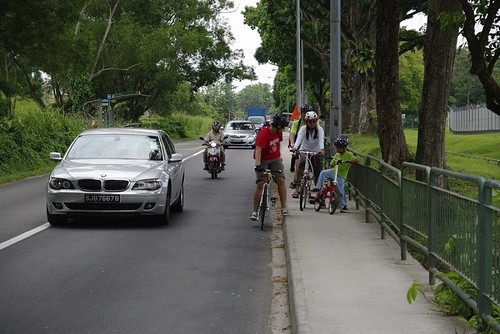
xmin=304 ymin=111 xmax=318 ymax=121
xmin=272 ymin=114 xmax=290 ymax=128
xmin=334 ymin=136 xmax=349 ymax=148
xmin=299 ymin=104 xmax=314 ymax=113
xmin=212 ymin=121 xmax=220 ymax=133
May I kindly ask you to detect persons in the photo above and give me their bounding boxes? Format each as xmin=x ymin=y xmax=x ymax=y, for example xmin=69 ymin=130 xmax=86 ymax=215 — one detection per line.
xmin=288 ymin=104 xmax=325 ymax=200
xmin=203 ymin=121 xmax=226 ymax=170
xmin=289 ymin=111 xmax=326 ymax=206
xmin=249 ymin=114 xmax=291 ymax=220
xmin=309 ymin=137 xmax=360 ymax=211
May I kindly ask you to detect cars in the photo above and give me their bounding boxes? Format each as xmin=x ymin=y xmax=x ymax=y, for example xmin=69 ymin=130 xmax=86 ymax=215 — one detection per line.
xmin=44 ymin=127 xmax=186 ymax=224
xmin=220 ymin=120 xmax=260 ymax=149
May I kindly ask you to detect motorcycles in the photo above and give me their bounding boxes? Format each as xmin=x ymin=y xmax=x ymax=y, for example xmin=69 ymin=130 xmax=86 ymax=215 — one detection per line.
xmin=200 ymin=136 xmax=229 ymax=179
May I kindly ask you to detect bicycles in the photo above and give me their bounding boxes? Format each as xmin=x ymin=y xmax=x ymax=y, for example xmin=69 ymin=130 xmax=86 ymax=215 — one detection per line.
xmin=254 ymin=166 xmax=283 ymax=230
xmin=313 ymin=155 xmax=352 ymax=215
xmin=288 ymin=144 xmax=325 ymax=211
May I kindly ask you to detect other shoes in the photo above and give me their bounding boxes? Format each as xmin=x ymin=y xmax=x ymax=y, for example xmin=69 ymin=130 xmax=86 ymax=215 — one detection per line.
xmin=221 ymin=166 xmax=225 ymax=171
xmin=289 ymin=180 xmax=297 ymax=189
xmin=281 ymin=208 xmax=290 ymax=216
xmin=203 ymin=166 xmax=209 ymax=170
xmin=292 ymin=191 xmax=301 ymax=198
xmin=250 ymin=211 xmax=257 ymax=221
xmin=309 ymin=188 xmax=320 ymax=193
xmin=341 ymin=207 xmax=348 ymax=212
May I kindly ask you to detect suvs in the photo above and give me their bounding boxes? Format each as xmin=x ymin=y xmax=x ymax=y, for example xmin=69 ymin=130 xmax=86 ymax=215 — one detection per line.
xmin=248 ymin=115 xmax=266 ymax=133
